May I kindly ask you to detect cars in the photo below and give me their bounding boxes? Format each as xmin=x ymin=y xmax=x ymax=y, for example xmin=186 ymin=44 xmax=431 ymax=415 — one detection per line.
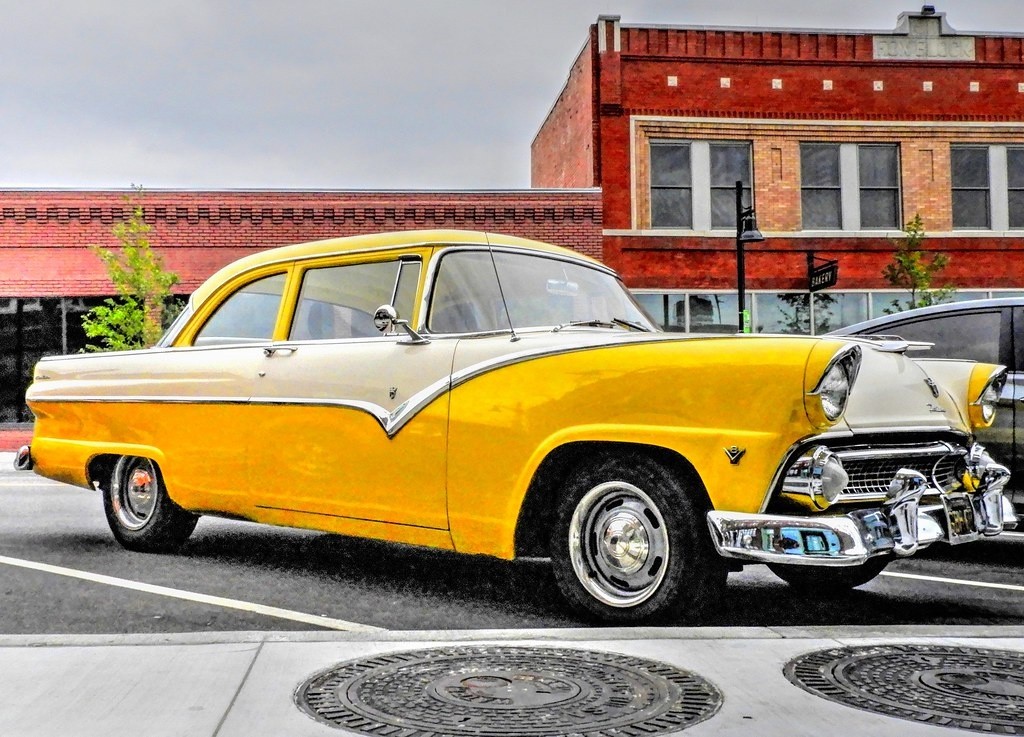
xmin=814 ymin=296 xmax=1024 ymax=547
xmin=13 ymin=226 xmax=1020 ymax=626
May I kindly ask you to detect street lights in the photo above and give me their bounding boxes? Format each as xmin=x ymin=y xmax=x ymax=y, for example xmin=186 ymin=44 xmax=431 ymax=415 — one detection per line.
xmin=735 ymin=179 xmax=765 ymax=334
